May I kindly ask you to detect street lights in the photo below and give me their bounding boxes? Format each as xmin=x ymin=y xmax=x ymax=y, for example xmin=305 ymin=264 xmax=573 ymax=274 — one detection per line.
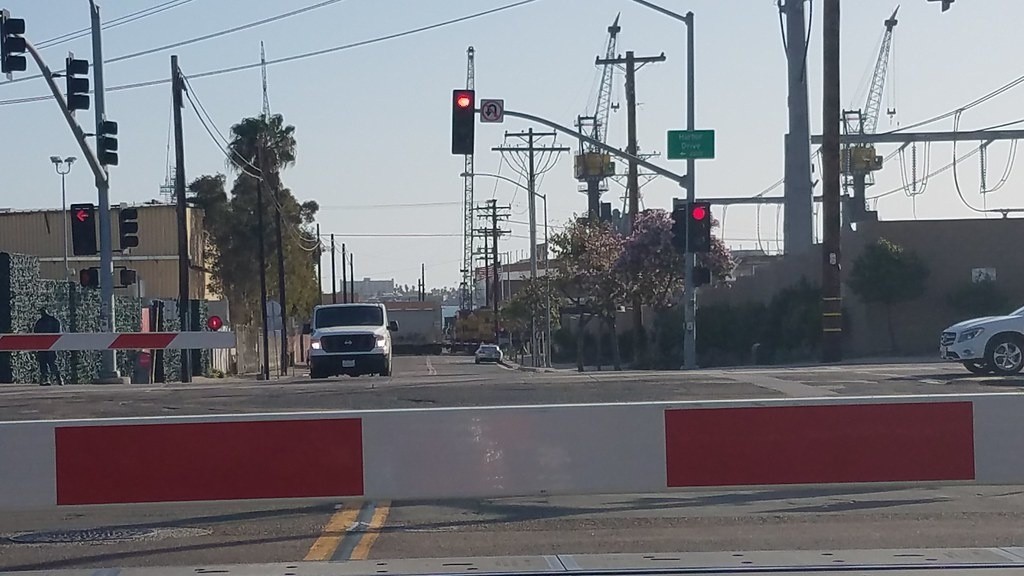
xmin=50 ymin=155 xmax=78 ymax=281
xmin=460 ymin=170 xmax=553 ymax=369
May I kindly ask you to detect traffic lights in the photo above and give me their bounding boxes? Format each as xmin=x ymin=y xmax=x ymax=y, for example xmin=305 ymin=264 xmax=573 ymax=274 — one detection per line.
xmin=2 ymin=8 xmax=27 ymax=76
xmin=695 ymin=267 xmax=712 ymax=285
xmin=80 ymin=268 xmax=98 ymax=290
xmin=66 ymin=50 xmax=91 ymax=112
xmin=671 ymin=199 xmax=686 ymax=254
xmin=101 ymin=121 xmax=120 ymax=167
xmin=117 ymin=207 xmax=139 ymax=247
xmin=71 ymin=203 xmax=97 ymax=256
xmin=451 ymin=89 xmax=476 ymax=156
xmin=688 ymin=202 xmax=712 ymax=253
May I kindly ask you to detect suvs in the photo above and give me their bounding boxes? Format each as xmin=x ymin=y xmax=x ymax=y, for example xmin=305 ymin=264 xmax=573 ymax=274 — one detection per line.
xmin=300 ymin=304 xmax=399 ymax=378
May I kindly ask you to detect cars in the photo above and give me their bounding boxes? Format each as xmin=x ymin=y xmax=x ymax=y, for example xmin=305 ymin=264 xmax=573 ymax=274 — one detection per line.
xmin=938 ymin=303 xmax=1023 ymax=378
xmin=476 ymin=345 xmax=504 ymax=366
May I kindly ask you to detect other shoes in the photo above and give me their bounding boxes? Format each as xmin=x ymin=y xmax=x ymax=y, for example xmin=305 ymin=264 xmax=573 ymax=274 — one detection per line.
xmin=40 ymin=382 xmax=52 ymax=386
xmin=59 ymin=381 xmax=64 ymax=386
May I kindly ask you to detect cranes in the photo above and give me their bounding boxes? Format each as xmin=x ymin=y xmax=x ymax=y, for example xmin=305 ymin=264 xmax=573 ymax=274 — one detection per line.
xmin=830 ymin=4 xmax=906 ymax=213
xmin=572 ymin=11 xmax=640 ymax=220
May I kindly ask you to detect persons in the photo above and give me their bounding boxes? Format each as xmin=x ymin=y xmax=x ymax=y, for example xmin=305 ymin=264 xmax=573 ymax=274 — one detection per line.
xmin=32 ymin=307 xmax=64 ymax=385
